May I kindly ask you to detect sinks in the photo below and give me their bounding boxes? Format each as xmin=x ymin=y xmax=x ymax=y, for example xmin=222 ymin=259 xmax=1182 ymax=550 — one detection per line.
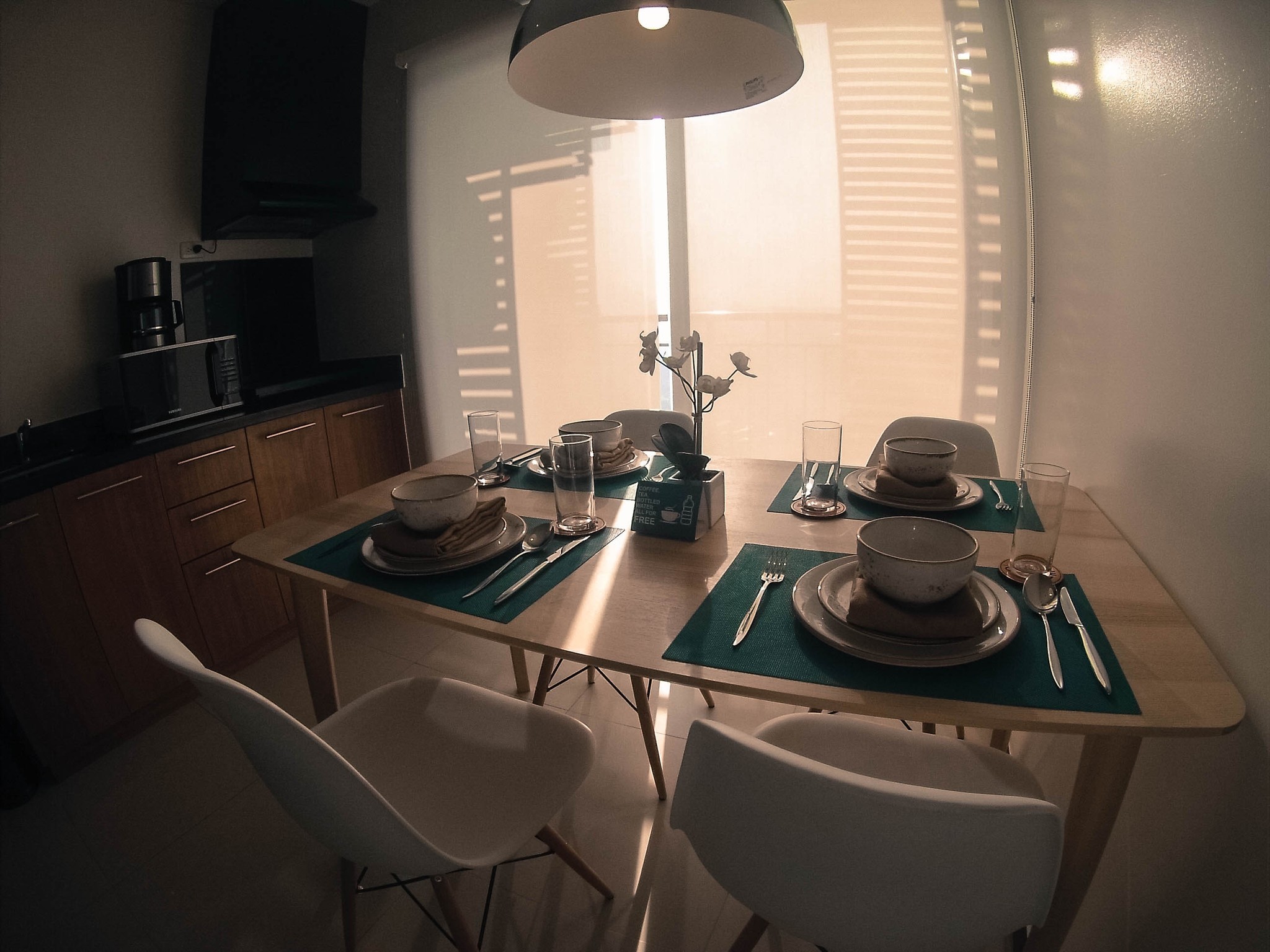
xmin=0 ymin=447 xmax=86 ymax=482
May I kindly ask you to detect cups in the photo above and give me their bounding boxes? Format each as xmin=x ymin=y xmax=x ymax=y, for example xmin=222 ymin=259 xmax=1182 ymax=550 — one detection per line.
xmin=1008 ymin=463 xmax=1070 ymax=579
xmin=800 ymin=421 xmax=842 ymax=513
xmin=467 ymin=410 xmax=504 ymax=483
xmin=549 ymin=434 xmax=596 ymax=532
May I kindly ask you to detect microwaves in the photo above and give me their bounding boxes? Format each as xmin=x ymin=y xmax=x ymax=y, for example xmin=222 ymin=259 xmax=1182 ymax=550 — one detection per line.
xmin=95 ymin=334 xmax=245 ymax=444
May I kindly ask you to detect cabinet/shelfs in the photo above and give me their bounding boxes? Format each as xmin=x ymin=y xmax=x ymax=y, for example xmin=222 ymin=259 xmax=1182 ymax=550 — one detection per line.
xmin=1 ymin=392 xmax=398 ymax=785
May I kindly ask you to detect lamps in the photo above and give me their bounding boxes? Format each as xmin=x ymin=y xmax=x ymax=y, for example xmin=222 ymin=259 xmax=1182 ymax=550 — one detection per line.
xmin=508 ymin=0 xmax=804 ymax=120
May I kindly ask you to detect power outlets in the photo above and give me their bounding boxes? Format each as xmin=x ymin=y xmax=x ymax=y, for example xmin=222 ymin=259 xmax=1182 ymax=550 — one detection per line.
xmin=180 ymin=242 xmax=205 ymax=259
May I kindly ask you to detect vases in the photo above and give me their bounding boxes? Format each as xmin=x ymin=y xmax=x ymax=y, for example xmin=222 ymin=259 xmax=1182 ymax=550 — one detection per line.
xmin=668 ymin=469 xmax=724 ymax=527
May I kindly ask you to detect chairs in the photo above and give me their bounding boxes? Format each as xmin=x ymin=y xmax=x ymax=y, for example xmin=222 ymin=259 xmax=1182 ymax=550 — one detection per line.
xmin=532 ymin=410 xmax=715 ymax=802
xmin=865 ymin=416 xmax=1001 ymax=478
xmin=134 ymin=617 xmax=615 ymax=952
xmin=669 ymin=711 xmax=1062 ymax=952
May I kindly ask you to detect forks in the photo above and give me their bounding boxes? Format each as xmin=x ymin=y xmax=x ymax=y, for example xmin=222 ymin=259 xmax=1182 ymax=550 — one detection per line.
xmin=989 ymin=479 xmax=1014 ymax=510
xmin=640 ymin=465 xmax=673 ymax=483
xmin=733 ymin=551 xmax=788 ymax=645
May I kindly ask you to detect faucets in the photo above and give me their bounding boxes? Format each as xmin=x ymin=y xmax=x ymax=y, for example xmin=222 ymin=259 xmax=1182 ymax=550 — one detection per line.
xmin=14 ymin=418 xmax=32 ymax=464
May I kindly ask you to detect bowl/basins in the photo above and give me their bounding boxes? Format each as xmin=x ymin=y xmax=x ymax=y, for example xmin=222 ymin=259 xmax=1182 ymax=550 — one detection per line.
xmin=856 ymin=516 xmax=979 ymax=606
xmin=559 ymin=420 xmax=622 ymax=452
xmin=884 ymin=437 xmax=958 ymax=485
xmin=391 ymin=474 xmax=478 ymax=534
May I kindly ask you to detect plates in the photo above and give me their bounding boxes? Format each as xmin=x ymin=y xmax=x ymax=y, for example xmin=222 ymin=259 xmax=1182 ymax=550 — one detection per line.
xmin=527 ymin=448 xmax=649 ymax=479
xmin=360 ymin=510 xmax=527 ymax=576
xmin=791 ymin=555 xmax=1020 ymax=667
xmin=844 ymin=466 xmax=984 ymax=511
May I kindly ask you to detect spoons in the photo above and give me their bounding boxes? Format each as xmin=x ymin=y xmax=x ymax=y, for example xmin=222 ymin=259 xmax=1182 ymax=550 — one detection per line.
xmin=1022 ymin=573 xmax=1064 ymax=689
xmin=461 ymin=522 xmax=555 ymax=599
xmin=496 ymin=455 xmax=540 ymax=468
xmin=815 ymin=463 xmax=835 ymax=490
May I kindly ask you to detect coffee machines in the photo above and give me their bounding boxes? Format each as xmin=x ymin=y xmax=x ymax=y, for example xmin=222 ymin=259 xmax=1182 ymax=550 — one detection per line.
xmin=114 ymin=257 xmax=184 ymax=353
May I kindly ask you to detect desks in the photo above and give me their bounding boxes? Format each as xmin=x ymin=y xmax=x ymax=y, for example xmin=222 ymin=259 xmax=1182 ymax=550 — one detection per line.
xmin=230 ymin=435 xmax=1244 ymax=952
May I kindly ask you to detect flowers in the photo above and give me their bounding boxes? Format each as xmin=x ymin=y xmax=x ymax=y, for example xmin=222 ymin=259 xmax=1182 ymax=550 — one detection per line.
xmin=639 ymin=325 xmax=757 ymax=454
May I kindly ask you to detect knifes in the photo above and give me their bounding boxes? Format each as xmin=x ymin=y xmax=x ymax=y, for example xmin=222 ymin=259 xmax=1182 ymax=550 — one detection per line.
xmin=792 ymin=462 xmax=818 ymax=501
xmin=482 ymin=447 xmax=543 ymax=472
xmin=1059 ymin=587 xmax=1112 ymax=696
xmin=492 ymin=536 xmax=591 ymax=606
